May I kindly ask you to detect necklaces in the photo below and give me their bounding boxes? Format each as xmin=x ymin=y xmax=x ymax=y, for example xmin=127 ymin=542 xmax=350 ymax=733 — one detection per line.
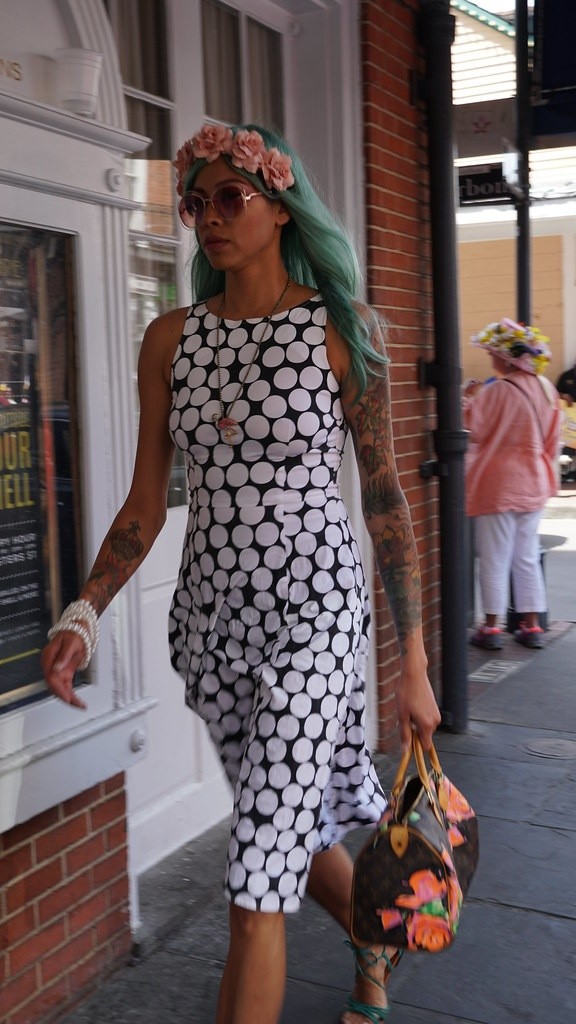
xmin=212 ymin=272 xmax=292 ymax=447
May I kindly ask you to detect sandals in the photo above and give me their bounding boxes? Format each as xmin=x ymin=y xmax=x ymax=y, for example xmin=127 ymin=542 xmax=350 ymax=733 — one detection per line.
xmin=341 ymin=938 xmax=405 ymax=1023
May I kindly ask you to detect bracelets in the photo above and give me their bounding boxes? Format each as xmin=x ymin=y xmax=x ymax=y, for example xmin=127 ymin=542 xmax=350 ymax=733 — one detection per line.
xmin=48 ymin=600 xmax=100 ymax=671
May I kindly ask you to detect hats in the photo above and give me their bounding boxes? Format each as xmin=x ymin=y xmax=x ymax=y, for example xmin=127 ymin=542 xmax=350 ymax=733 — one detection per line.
xmin=469 ymin=318 xmax=552 ymax=375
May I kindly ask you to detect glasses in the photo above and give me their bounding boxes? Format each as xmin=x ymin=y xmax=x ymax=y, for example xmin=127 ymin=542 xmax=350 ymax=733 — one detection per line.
xmin=178 ymin=184 xmax=263 ymax=229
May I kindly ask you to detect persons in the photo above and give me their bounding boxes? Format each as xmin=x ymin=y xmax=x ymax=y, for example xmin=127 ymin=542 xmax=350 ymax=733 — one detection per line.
xmin=557 ymin=368 xmax=576 ymax=482
xmin=42 ymin=124 xmax=442 ymax=1024
xmin=463 ymin=320 xmax=561 ymax=648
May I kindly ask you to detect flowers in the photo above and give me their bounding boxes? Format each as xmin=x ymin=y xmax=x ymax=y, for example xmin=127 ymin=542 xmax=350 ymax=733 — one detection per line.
xmin=468 ymin=320 xmax=552 ymax=374
xmin=165 ymin=123 xmax=295 ymax=192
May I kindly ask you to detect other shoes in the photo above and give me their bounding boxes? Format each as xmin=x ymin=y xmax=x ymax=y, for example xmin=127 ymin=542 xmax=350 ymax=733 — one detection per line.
xmin=515 ymin=627 xmax=544 ymax=647
xmin=470 ymin=627 xmax=504 ymax=651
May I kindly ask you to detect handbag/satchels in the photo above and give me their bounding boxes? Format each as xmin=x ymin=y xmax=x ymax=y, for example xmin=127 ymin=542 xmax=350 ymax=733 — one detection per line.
xmin=350 ymin=726 xmax=480 ymax=954
